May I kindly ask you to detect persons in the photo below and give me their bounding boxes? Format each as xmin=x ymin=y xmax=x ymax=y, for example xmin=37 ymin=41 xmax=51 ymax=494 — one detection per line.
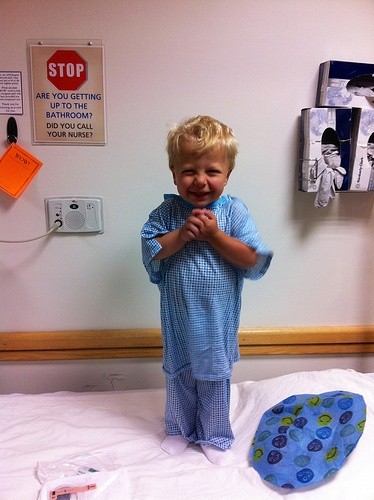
xmin=140 ymin=116 xmax=274 ymax=464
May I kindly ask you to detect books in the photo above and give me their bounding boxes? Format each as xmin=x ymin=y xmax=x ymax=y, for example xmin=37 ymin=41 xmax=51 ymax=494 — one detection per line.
xmin=0 ymin=142 xmax=44 ymax=199
